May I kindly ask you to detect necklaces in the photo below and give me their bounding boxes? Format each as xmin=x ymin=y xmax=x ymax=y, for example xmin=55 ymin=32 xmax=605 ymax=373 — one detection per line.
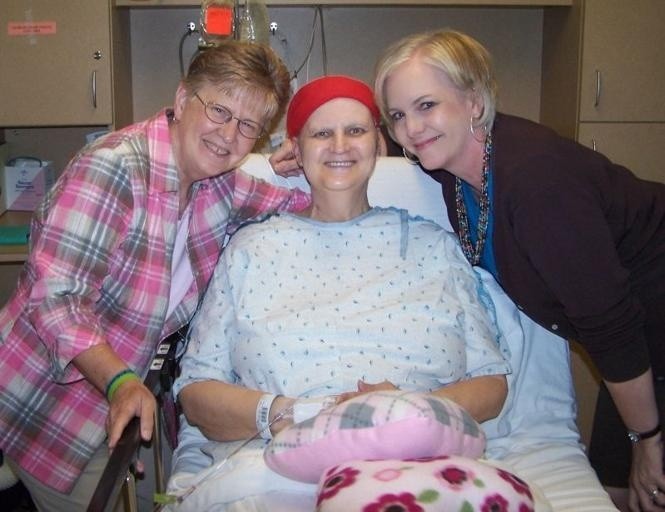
xmin=457 ymin=142 xmax=492 ymax=268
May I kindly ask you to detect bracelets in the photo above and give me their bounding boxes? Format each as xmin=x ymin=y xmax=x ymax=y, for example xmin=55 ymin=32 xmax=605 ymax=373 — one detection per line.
xmin=105 ymin=370 xmax=136 ymax=403
xmin=256 ymin=393 xmax=281 ymax=441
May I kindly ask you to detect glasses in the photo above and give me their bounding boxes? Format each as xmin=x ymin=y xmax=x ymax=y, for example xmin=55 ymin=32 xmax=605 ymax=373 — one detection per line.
xmin=188 ymin=87 xmax=265 ymax=142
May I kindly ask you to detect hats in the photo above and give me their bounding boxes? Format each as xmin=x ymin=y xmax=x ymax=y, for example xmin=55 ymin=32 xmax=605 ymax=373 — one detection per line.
xmin=284 ymin=73 xmax=380 ymax=141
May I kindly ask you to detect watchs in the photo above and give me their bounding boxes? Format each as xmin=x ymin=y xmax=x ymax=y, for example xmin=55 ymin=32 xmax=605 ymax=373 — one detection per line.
xmin=625 ymin=421 xmax=662 ymax=443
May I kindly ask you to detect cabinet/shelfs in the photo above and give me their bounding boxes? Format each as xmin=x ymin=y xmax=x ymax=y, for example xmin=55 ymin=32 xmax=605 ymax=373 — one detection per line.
xmin=1 ymin=210 xmax=37 ymax=303
xmin=540 ymin=1 xmax=665 ymax=191
xmin=2 ymin=1 xmax=132 ymax=127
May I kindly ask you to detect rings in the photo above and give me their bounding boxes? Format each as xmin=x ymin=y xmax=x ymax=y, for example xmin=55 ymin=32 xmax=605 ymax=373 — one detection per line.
xmin=647 ymin=489 xmax=660 ymax=497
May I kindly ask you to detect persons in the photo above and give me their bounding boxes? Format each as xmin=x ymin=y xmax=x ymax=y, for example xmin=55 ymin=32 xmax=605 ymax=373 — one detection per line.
xmin=1 ymin=41 xmax=312 ymax=511
xmin=174 ymin=75 xmax=512 ymax=441
xmin=269 ymin=33 xmax=664 ymax=511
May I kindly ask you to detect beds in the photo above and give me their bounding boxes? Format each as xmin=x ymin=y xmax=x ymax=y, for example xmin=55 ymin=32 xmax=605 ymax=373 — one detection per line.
xmin=91 ymin=153 xmax=625 ymax=511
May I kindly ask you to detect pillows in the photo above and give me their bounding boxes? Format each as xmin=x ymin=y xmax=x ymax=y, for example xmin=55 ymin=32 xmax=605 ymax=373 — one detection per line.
xmin=264 ymin=389 xmax=487 ymax=486
xmin=310 ymin=459 xmax=536 ymax=511
